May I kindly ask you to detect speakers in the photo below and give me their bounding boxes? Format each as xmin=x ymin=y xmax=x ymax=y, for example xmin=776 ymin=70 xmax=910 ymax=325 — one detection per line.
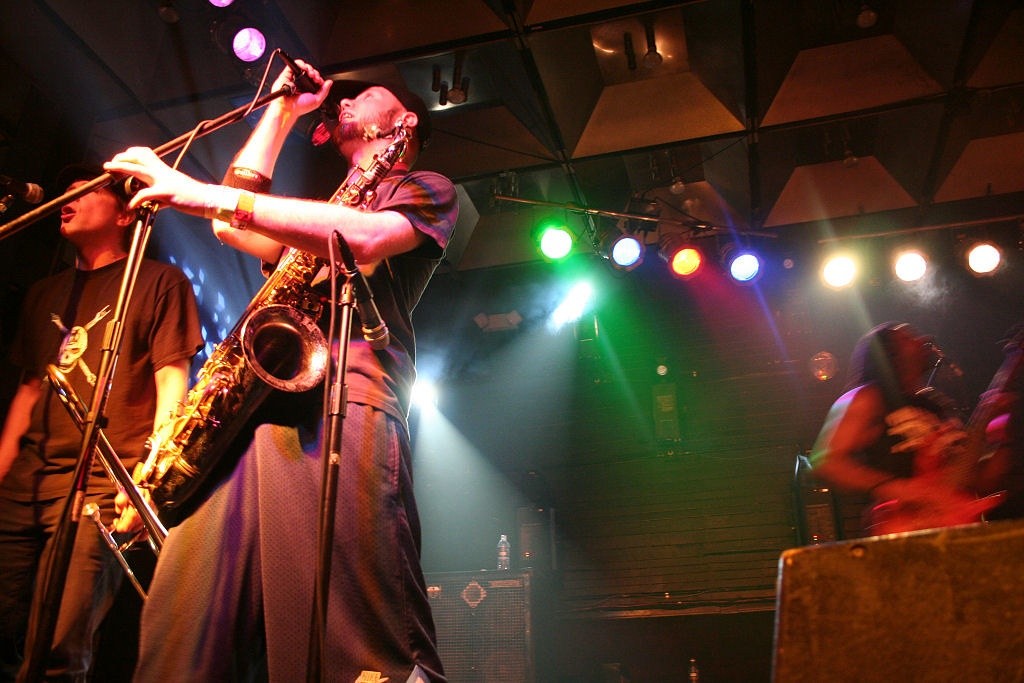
xmin=771 ymin=518 xmax=1024 ymax=683
xmin=422 ymin=571 xmax=539 ymax=682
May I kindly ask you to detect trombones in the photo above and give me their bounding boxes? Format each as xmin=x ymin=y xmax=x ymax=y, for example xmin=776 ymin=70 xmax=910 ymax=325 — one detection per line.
xmin=45 ymin=360 xmax=172 ymax=603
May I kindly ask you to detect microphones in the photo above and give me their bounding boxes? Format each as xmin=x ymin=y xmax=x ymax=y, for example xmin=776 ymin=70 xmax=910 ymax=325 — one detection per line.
xmin=277 ymin=47 xmax=342 ymax=122
xmin=0 ymin=175 xmax=44 ymax=204
xmin=337 ymin=236 xmax=390 ymax=349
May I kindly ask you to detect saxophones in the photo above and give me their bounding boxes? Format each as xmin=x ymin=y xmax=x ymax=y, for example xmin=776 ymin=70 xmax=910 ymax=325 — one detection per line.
xmin=131 ymin=122 xmax=411 ymax=512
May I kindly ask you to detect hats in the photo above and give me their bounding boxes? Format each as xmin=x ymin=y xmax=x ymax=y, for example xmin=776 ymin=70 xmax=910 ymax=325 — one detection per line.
xmin=322 ymin=79 xmax=432 ymax=149
xmin=57 ymin=162 xmax=155 ymax=230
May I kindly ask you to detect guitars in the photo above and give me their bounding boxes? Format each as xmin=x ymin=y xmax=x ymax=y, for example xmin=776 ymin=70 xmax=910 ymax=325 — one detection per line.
xmin=866 ymin=327 xmax=1024 ymax=537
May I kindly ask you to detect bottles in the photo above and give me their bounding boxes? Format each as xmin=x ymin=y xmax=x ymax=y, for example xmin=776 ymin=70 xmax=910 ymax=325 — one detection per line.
xmin=496 ymin=534 xmax=511 ymax=571
xmin=688 ymin=658 xmax=700 ymax=683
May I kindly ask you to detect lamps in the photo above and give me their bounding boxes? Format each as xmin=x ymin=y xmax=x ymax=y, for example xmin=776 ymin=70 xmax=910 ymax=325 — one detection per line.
xmin=956 ymin=235 xmax=1012 ymax=279
xmin=597 ymin=222 xmax=650 ymax=278
xmin=715 ymin=239 xmax=767 ymax=291
xmin=656 ymin=229 xmax=707 ymax=282
xmin=203 ymin=13 xmax=269 ymax=66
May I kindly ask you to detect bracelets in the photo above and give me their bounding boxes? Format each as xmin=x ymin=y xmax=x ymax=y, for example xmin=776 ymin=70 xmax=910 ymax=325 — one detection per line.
xmin=230 ymin=190 xmax=255 ymax=231
xmin=867 ymin=475 xmax=902 ymax=495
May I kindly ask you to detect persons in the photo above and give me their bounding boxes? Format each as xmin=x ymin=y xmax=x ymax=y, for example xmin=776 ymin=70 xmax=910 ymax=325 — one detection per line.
xmin=0 ymin=169 xmax=205 ymax=683
xmin=104 ymin=59 xmax=459 ymax=683
xmin=807 ymin=321 xmax=1024 ymax=541
